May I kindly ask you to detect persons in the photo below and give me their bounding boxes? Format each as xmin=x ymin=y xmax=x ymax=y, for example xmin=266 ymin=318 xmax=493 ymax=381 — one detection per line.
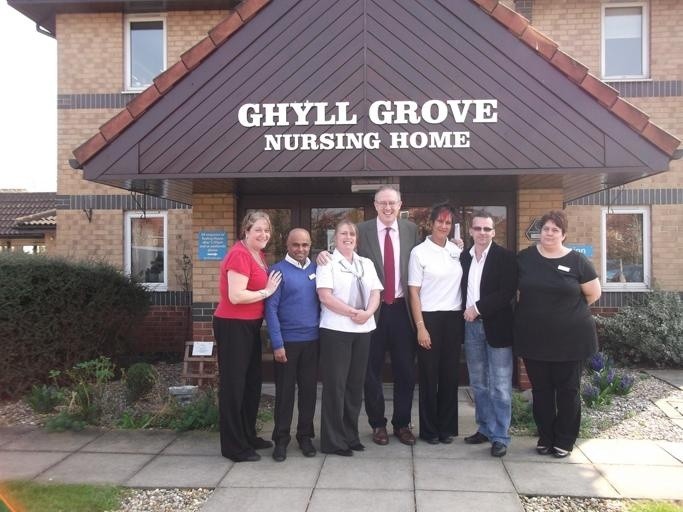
xmin=211 ymin=208 xmax=283 ymax=462
xmin=265 ymin=227 xmax=320 ymax=462
xmin=516 ymin=210 xmax=602 ymax=458
xmin=315 ymin=218 xmax=385 ymax=456
xmin=407 ymin=203 xmax=465 ymax=444
xmin=315 ymin=184 xmax=465 ymax=444
xmin=459 ymin=211 xmax=515 ymax=457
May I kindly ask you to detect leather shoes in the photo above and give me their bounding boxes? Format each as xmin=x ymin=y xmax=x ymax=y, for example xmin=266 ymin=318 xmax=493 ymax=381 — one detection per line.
xmin=492 ymin=441 xmax=506 ymax=455
xmin=393 ymin=427 xmax=416 ymax=444
xmin=243 ymin=453 xmax=261 ymax=462
xmin=298 ymin=438 xmax=316 ymax=457
xmin=274 ymin=445 xmax=285 ymax=462
xmin=465 ymin=432 xmax=488 ymax=443
xmin=336 ymin=444 xmax=365 ymax=457
xmin=427 ymin=434 xmax=453 ymax=444
xmin=257 ymin=437 xmax=272 ymax=449
xmin=373 ymin=426 xmax=388 ymax=445
xmin=537 ymin=445 xmax=570 ymax=457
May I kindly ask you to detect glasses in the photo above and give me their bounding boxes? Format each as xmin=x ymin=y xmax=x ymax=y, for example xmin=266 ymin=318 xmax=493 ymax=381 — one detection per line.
xmin=472 ymin=227 xmax=493 ymax=232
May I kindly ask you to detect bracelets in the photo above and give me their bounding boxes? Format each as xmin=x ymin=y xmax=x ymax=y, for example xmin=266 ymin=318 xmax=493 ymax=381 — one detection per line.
xmin=259 ymin=290 xmax=267 ymax=299
xmin=416 ymin=320 xmax=424 ymax=326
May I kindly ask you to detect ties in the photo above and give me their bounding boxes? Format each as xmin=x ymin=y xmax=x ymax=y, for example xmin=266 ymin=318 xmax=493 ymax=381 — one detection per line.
xmin=384 ymin=227 xmax=395 ymax=305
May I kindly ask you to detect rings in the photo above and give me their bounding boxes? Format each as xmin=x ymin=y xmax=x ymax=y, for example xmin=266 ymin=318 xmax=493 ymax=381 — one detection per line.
xmin=421 ymin=344 xmax=424 ymax=345
